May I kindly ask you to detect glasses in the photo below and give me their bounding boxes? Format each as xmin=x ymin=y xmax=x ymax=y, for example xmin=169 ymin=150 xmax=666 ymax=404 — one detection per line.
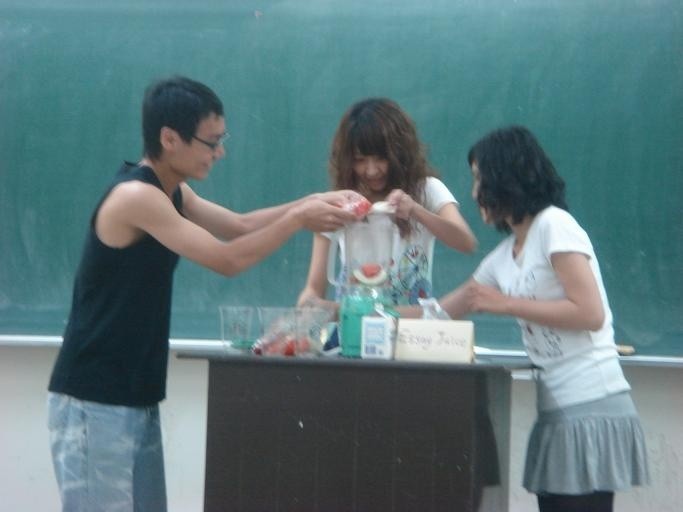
xmin=191 ymin=133 xmax=230 ymax=149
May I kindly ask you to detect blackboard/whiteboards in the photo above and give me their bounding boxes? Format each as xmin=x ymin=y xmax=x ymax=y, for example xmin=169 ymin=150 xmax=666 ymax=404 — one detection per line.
xmin=0 ymin=0 xmax=683 ymax=367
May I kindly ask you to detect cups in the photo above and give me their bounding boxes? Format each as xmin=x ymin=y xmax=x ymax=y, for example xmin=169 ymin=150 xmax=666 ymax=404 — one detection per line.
xmin=291 ymin=306 xmax=329 ymax=361
xmin=218 ymin=304 xmax=254 ymax=356
xmin=256 ymin=306 xmax=294 ymax=359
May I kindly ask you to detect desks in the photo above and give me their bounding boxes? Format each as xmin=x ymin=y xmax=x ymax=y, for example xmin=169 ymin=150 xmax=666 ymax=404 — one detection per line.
xmin=174 ymin=349 xmax=512 ymax=512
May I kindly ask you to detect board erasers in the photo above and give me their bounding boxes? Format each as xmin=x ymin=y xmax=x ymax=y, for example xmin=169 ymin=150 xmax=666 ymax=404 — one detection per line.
xmin=616 ymin=345 xmax=634 ymax=354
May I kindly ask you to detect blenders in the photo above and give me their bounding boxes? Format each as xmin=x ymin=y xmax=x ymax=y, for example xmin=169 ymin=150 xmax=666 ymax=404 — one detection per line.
xmin=325 ymin=209 xmax=401 ymax=360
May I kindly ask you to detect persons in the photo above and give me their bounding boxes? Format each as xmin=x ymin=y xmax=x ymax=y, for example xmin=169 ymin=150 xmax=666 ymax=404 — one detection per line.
xmin=46 ymin=74 xmax=371 ymax=511
xmin=308 ymin=123 xmax=652 ymax=511
xmin=295 ymin=95 xmax=477 ymax=310
